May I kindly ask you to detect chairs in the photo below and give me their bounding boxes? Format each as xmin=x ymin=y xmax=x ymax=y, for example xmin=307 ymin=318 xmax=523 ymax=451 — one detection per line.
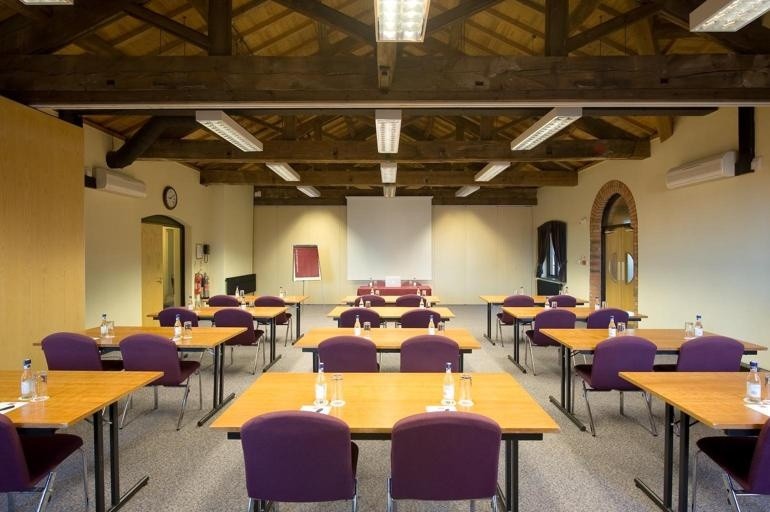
xmin=41 ymin=332 xmax=113 ymax=426
xmin=255 ymin=296 xmax=292 ymax=347
xmin=401 ymin=309 xmax=442 ymax=328
xmin=587 ymin=308 xmax=629 ymax=328
xmin=675 ymin=336 xmax=744 ymax=372
xmin=318 ymin=336 xmax=379 ymax=373
xmin=159 ymin=308 xmax=215 ymax=375
xmin=118 ymin=333 xmax=202 ymax=431
xmin=396 ymin=294 xmax=433 ymax=307
xmin=208 ymin=295 xmax=239 ymax=327
xmin=692 ymin=416 xmax=770 ymax=512
xmin=240 ymin=411 xmax=359 ymax=512
xmin=213 ymin=309 xmax=266 ymax=375
xmin=386 ymin=411 xmax=502 ymax=512
xmin=400 ymin=335 xmax=460 ymax=373
xmin=369 ymin=280 xmax=385 ymax=286
xmin=0 ymin=414 xmax=90 ymax=512
xmin=401 ymin=281 xmax=422 ymax=286
xmin=548 ymin=295 xmax=576 ymax=306
xmin=340 ymin=308 xmax=380 ymax=328
xmin=355 ymin=295 xmax=385 ymax=307
xmin=525 ymin=309 xmax=576 ymax=376
xmin=572 ymin=336 xmax=657 ymax=438
xmin=496 ymin=295 xmax=534 ymax=347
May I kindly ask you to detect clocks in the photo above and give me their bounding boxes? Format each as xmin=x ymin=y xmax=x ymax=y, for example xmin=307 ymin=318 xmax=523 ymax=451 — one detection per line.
xmin=163 ymin=186 xmax=177 ymax=210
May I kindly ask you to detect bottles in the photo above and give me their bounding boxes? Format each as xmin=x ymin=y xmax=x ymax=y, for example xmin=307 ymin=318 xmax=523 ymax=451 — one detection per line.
xmin=694 ymin=312 xmax=704 ymax=337
xmin=370 ymin=287 xmax=374 ymax=295
xmin=359 ymin=296 xmax=364 ymax=307
xmin=746 ymin=361 xmax=762 ymax=404
xmin=100 ymin=313 xmax=107 ymax=337
xmin=354 ymin=315 xmax=361 ymax=337
xmin=594 ymin=296 xmax=600 ymax=311
xmin=188 ymin=295 xmax=193 ymax=311
xmin=426 ymin=300 xmax=431 ymax=309
xmin=235 ymin=285 xmax=240 ymax=298
xmin=441 ymin=362 xmax=457 ymax=406
xmin=240 ymin=297 xmax=246 ymax=310
xmin=419 ymin=297 xmax=425 ymax=308
xmin=313 ymin=363 xmax=329 ymax=406
xmin=416 ymin=287 xmax=420 ymax=296
xmin=608 ymin=315 xmax=616 ymax=338
xmin=20 ymin=359 xmax=36 ymax=400
xmin=279 ymin=287 xmax=283 ymax=298
xmin=428 ymin=314 xmax=436 ymax=336
xmin=174 ymin=314 xmax=182 ymax=339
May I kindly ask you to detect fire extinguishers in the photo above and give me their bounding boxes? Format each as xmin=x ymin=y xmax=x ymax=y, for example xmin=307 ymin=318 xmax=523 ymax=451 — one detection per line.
xmin=202 ymin=272 xmax=210 ymax=299
xmin=195 ymin=268 xmax=203 ymax=293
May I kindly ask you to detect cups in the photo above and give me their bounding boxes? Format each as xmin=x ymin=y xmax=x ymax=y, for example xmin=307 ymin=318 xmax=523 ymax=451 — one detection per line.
xmin=330 ymin=374 xmax=345 ymax=408
xmin=544 ymin=297 xmax=550 ymax=310
xmin=106 ymin=321 xmax=115 ymax=338
xmin=438 ymin=322 xmax=445 ymax=336
xmin=240 ymin=290 xmax=244 ymax=297
xmin=764 ymin=375 xmax=770 ymax=404
xmin=33 ymin=371 xmax=48 ymax=402
xmin=617 ymin=322 xmax=625 ymax=337
xmin=459 ymin=374 xmax=474 ymax=407
xmin=363 ymin=322 xmax=371 ymax=340
xmin=602 ymin=301 xmax=608 ymax=309
xmin=422 ymin=290 xmax=426 ymax=297
xmin=184 ymin=321 xmax=193 ymax=339
xmin=375 ymin=290 xmax=379 ymax=296
xmin=248 ymin=300 xmax=255 ymax=312
xmin=685 ymin=321 xmax=694 ymax=338
xmin=552 ymin=301 xmax=557 ymax=309
xmin=366 ymin=301 xmax=371 ymax=309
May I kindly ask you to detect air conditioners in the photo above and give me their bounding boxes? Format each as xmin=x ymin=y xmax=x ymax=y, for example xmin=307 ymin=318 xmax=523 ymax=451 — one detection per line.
xmin=665 ymin=149 xmax=737 ymax=190
xmin=94 ymin=167 xmax=146 ymax=199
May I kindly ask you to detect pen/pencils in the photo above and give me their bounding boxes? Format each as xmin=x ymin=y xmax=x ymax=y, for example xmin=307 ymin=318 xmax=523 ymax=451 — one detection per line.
xmin=0 ymin=406 xmax=15 ymax=411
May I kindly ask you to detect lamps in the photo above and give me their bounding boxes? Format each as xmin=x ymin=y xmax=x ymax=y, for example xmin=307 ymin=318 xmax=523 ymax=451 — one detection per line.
xmin=195 ymin=110 xmax=263 ymax=152
xmin=265 ymin=161 xmax=301 ymax=181
xmin=689 ymin=0 xmax=770 ymax=32
xmin=375 ymin=109 xmax=402 ymax=153
xmin=383 ymin=185 xmax=396 ymax=198
xmin=296 ymin=186 xmax=321 ymax=197
xmin=374 ymin=0 xmax=430 ymax=43
xmin=380 ymin=161 xmax=397 ymax=183
xmin=455 ymin=183 xmax=480 ymax=197
xmin=474 ymin=160 xmax=510 ymax=182
xmin=510 ymin=107 xmax=583 ymax=151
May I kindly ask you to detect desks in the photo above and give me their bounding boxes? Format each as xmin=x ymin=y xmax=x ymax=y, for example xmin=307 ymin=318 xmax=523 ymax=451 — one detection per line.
xmin=357 ymin=286 xmax=431 ymax=296
xmin=209 ymin=372 xmax=561 ymax=511
xmin=539 ymin=328 xmax=768 ymax=433
xmin=32 ymin=327 xmax=248 ymax=427
xmin=145 ymin=307 xmax=288 ymax=373
xmin=202 ymin=295 xmax=311 ymax=346
xmin=326 ymin=306 xmax=455 ymax=328
xmin=341 ymin=295 xmax=440 ymax=307
xmin=0 ymin=370 xmax=164 ymax=512
xmin=293 ymin=328 xmax=482 ymax=372
xmin=618 ymin=371 xmax=769 ymax=512
xmin=479 ymin=295 xmax=590 ymax=346
xmin=501 ymin=307 xmax=648 ymax=374
